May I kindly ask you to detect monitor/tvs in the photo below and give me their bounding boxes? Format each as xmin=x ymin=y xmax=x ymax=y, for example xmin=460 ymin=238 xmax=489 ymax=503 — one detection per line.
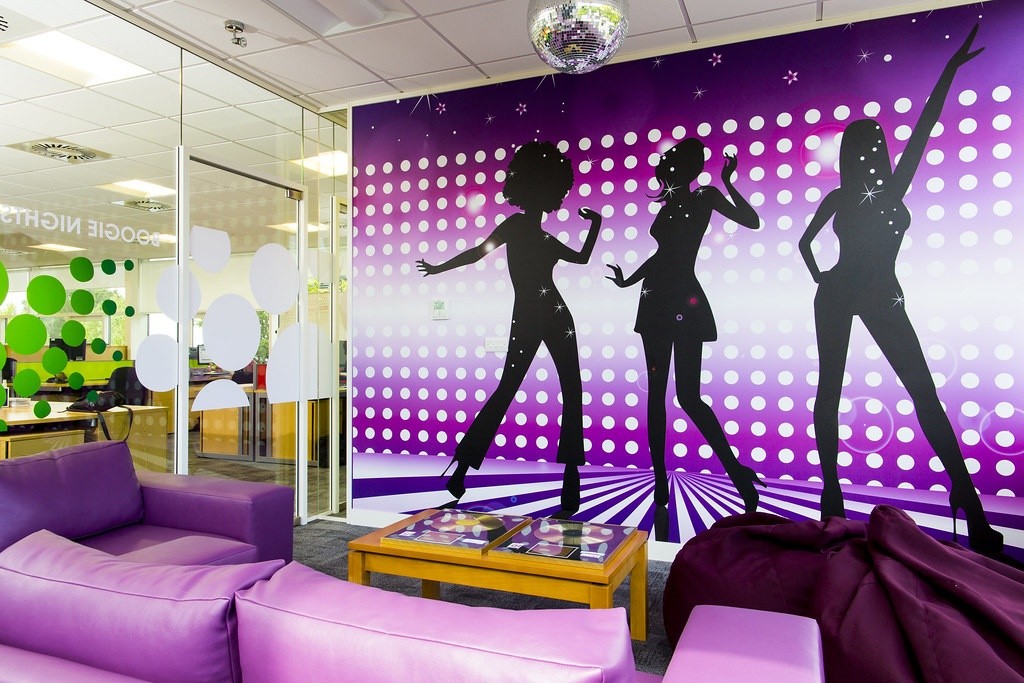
xmin=197 ymin=345 xmax=215 ymax=365
xmin=49 ymin=338 xmax=86 ymax=361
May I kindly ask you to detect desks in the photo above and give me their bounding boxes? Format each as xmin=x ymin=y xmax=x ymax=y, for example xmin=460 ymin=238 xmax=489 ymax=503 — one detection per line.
xmin=16 ymin=378 xmax=109 ymax=401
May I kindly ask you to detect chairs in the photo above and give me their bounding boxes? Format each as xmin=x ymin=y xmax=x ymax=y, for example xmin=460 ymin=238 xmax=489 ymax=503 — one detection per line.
xmin=104 ymin=367 xmax=149 ymax=406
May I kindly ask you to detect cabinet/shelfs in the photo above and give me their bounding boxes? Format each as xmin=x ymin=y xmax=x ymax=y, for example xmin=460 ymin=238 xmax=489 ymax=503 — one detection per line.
xmin=194 ymin=386 xmax=256 ymax=461
xmin=0 ymin=401 xmax=170 ymax=473
xmin=254 ymin=385 xmax=346 ymax=467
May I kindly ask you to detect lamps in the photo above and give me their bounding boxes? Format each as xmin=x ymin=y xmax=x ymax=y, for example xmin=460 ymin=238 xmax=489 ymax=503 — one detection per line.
xmin=225 ymin=20 xmax=248 ymax=48
xmin=526 ymin=0 xmax=630 ymax=74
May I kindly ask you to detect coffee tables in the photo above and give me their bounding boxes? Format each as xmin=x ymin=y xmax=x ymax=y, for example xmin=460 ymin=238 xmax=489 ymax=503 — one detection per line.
xmin=347 ymin=507 xmax=650 ymax=642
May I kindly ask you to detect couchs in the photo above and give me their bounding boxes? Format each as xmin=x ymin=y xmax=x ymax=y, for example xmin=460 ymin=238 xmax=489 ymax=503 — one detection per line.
xmin=0 ymin=439 xmax=294 ymax=566
xmin=0 ymin=528 xmax=824 ymax=683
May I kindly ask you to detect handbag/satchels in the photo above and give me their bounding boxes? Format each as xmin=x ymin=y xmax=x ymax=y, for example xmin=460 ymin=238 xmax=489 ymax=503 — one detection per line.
xmin=68 ymin=391 xmax=133 ymax=442
